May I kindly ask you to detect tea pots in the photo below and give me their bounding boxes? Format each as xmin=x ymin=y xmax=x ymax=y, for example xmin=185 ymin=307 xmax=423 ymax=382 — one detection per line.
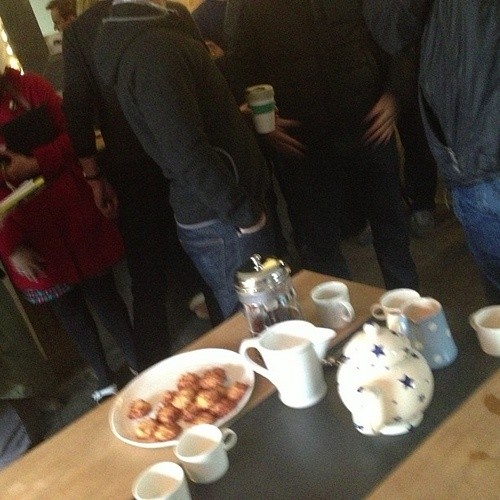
xmin=336 ymin=323 xmax=436 ymax=439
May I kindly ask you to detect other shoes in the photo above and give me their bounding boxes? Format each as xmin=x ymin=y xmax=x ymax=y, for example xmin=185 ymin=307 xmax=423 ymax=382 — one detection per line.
xmin=92 ymin=383 xmax=119 ymax=405
xmin=188 ymin=292 xmax=210 ymax=321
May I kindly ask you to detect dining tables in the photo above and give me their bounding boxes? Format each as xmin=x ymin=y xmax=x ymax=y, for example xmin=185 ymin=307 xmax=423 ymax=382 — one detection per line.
xmin=1 ymin=270 xmax=500 ymax=500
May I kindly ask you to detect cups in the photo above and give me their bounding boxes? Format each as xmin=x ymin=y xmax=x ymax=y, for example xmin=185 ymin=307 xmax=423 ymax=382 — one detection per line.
xmin=173 ymin=423 xmax=237 ymax=484
xmin=311 ymin=281 xmax=355 ymax=329
xmin=245 ymin=84 xmax=276 ymax=134
xmin=370 ymin=288 xmax=420 ymax=336
xmin=131 ymin=461 xmax=192 ymax=500
xmin=469 ymin=304 xmax=499 ymax=358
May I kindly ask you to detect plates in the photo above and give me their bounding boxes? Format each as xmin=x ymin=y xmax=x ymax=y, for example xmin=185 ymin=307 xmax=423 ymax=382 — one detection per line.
xmin=109 ymin=348 xmax=255 ymax=448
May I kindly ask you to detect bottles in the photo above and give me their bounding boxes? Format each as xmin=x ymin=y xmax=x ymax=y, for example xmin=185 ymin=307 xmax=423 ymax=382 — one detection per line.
xmin=233 ymin=253 xmax=304 ymax=361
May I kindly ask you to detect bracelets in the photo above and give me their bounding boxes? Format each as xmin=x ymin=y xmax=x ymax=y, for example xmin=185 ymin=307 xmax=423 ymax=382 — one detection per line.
xmin=83 ymin=171 xmax=102 ymax=180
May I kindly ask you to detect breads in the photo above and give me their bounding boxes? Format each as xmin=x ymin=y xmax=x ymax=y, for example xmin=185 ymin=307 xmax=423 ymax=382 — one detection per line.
xmin=127 ymin=367 xmax=248 ymax=444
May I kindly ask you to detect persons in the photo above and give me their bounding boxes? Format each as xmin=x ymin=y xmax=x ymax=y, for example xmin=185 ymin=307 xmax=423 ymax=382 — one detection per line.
xmin=47 ymin=0 xmax=282 ymax=361
xmin=192 ymin=0 xmax=500 ymax=302
xmin=0 ymin=62 xmax=147 ymax=405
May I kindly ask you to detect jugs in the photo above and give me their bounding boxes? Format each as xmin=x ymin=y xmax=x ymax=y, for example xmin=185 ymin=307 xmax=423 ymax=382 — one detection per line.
xmin=239 ymin=320 xmax=338 ymax=410
xmin=400 ymin=296 xmax=458 ymax=370
